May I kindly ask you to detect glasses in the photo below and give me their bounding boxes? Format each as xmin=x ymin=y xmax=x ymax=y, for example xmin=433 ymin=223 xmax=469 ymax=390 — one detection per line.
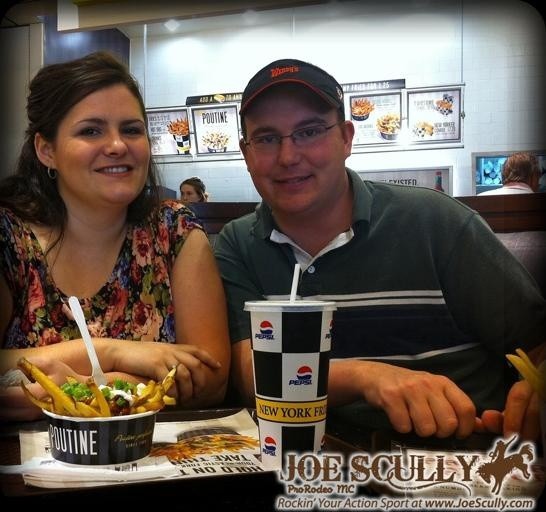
xmin=244 ymin=122 xmax=340 ymax=151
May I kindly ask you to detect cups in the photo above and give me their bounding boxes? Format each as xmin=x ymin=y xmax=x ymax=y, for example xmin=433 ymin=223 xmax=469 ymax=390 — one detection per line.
xmin=244 ymin=300 xmax=338 ymax=472
xmin=173 ymin=134 xmax=190 ymax=154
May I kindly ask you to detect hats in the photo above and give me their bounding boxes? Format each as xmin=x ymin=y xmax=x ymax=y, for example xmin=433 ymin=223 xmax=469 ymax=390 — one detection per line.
xmin=240 ymin=59 xmax=344 ymax=115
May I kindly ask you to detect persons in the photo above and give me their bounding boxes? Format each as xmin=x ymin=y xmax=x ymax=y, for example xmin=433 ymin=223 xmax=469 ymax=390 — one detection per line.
xmin=0 ymin=50 xmax=232 ymax=424
xmin=214 ymin=60 xmax=545 ymax=454
xmin=178 ymin=175 xmax=208 ymax=207
xmin=476 ymin=151 xmax=541 ymax=198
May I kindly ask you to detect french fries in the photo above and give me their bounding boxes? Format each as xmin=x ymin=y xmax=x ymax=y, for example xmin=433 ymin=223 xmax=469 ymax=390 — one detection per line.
xmin=167 ymin=117 xmax=188 ymax=135
xmin=505 ymin=349 xmax=543 ymax=391
xmin=352 ymin=99 xmax=374 ymax=115
xmin=16 ymin=357 xmax=178 ymax=417
